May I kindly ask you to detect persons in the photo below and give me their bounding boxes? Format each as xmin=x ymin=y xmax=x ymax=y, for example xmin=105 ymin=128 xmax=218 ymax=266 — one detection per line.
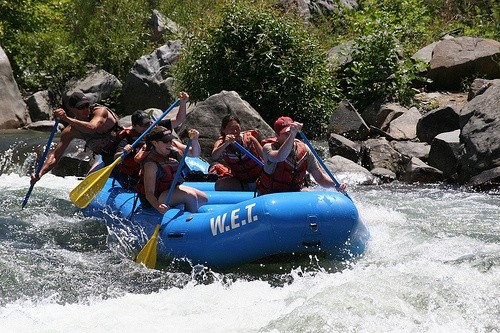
xmin=28 ymin=91 xmax=127 ymax=185
xmin=211 ymin=115 xmax=265 ymax=191
xmin=256 ymin=117 xmax=348 ymax=197
xmin=138 ymin=126 xmax=209 ymax=215
xmin=113 ymin=90 xmax=190 ymax=193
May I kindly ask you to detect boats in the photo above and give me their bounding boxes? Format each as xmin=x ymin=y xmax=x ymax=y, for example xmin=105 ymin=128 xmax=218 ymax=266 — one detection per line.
xmin=78 ymin=155 xmax=372 ymax=263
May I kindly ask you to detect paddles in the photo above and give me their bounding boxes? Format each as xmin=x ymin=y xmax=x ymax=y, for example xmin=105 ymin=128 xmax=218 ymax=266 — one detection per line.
xmin=137 ymin=135 xmax=193 ymax=269
xmin=232 ymin=139 xmax=264 ymax=167
xmin=299 ymin=129 xmax=354 ymax=203
xmin=69 ymin=97 xmax=182 ymax=208
xmin=22 ymin=114 xmax=62 ymax=207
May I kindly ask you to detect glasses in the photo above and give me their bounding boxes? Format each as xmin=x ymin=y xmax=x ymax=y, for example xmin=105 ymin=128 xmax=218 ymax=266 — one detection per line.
xmin=76 ymin=102 xmax=90 ymax=111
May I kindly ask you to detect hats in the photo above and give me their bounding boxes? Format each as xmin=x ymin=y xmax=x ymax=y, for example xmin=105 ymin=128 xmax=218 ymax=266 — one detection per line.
xmin=273 ymin=116 xmax=295 ymax=134
xmin=131 ymin=110 xmax=154 ymax=127
xmin=151 ymin=129 xmax=178 ymax=142
xmin=68 ymin=91 xmax=91 ymax=108
